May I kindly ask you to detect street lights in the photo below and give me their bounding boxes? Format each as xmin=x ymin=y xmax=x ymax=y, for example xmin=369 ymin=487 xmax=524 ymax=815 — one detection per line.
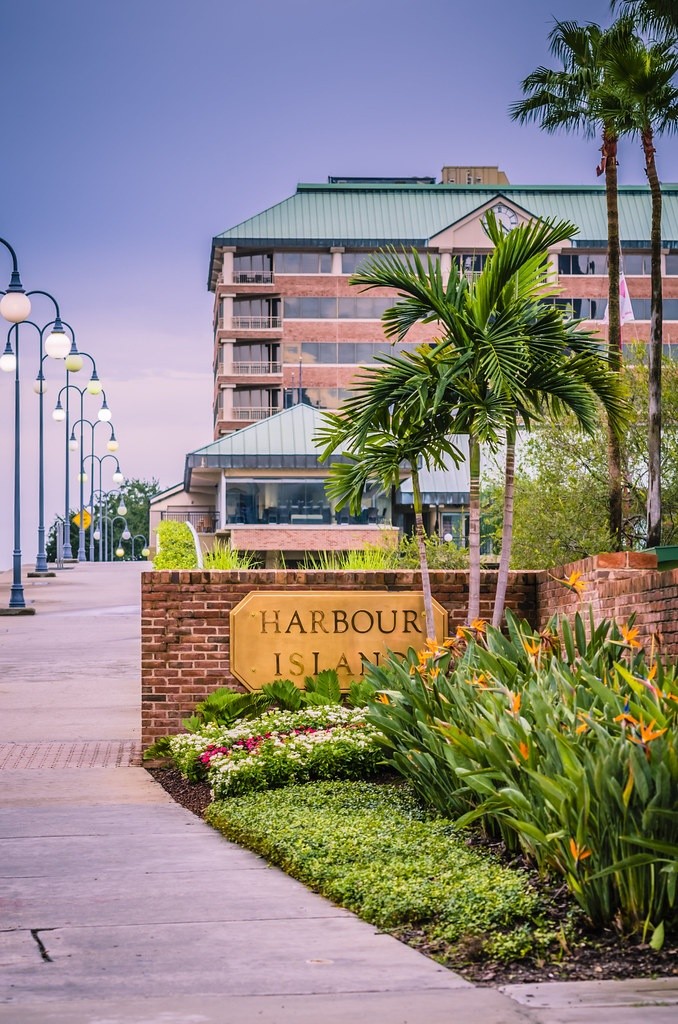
xmin=0 ymin=288 xmax=71 ymax=617
xmin=86 ymin=490 xmax=128 ymax=563
xmin=32 ymin=352 xmax=103 ymax=564
xmin=50 ymin=384 xmax=112 ymax=562
xmin=69 ymin=418 xmax=119 ymax=562
xmin=93 ymin=516 xmax=131 ymax=562
xmin=78 ymin=454 xmax=124 ymax=562
xmin=1 ymin=321 xmax=84 ymax=579
xmin=115 ymin=534 xmax=150 ymax=561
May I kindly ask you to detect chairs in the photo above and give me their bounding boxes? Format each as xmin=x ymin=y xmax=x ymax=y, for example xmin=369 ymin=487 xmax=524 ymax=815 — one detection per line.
xmin=367 ymin=506 xmax=378 ymax=524
xmin=235 ymin=503 xmax=247 ymax=524
xmin=378 ymin=508 xmax=387 ymax=523
xmin=267 ymin=506 xmax=278 ymax=524
xmin=336 ymin=507 xmax=350 ymax=524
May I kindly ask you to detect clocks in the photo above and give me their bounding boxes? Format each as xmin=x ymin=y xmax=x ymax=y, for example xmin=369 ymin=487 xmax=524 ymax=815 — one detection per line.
xmin=482 ymin=205 xmax=519 ymax=239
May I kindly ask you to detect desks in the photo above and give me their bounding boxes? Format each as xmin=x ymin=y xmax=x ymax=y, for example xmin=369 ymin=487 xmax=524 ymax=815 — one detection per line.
xmin=291 ymin=513 xmax=324 ymax=524
